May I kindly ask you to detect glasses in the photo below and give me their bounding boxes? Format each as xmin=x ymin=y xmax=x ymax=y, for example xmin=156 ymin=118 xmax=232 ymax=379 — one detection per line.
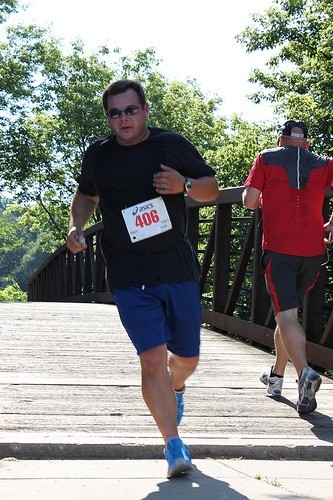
xmin=104 ymin=103 xmax=143 ymax=119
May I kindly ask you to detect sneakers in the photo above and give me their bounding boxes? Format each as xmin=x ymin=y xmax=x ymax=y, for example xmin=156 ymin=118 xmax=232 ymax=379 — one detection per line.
xmin=295 ymin=367 xmax=322 ymax=414
xmin=169 ymin=365 xmax=187 ymax=426
xmin=163 ymin=437 xmax=194 ymax=476
xmin=259 ymin=363 xmax=284 ymax=396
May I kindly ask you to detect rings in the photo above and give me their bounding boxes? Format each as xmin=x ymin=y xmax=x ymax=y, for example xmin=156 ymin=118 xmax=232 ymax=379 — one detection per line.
xmin=164 ymin=184 xmax=167 ymax=189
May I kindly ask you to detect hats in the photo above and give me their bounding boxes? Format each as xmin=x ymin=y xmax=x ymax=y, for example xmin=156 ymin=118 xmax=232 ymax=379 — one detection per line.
xmin=278 ymin=121 xmax=308 ymax=146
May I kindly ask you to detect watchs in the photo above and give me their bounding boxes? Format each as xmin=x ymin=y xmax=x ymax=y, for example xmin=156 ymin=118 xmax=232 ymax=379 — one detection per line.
xmin=184 ymin=175 xmax=192 ymax=197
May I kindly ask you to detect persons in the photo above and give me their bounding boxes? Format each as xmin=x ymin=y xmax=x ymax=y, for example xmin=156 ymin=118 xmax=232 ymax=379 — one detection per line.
xmin=66 ymin=81 xmax=220 ymax=477
xmin=242 ymin=119 xmax=333 ymax=414
